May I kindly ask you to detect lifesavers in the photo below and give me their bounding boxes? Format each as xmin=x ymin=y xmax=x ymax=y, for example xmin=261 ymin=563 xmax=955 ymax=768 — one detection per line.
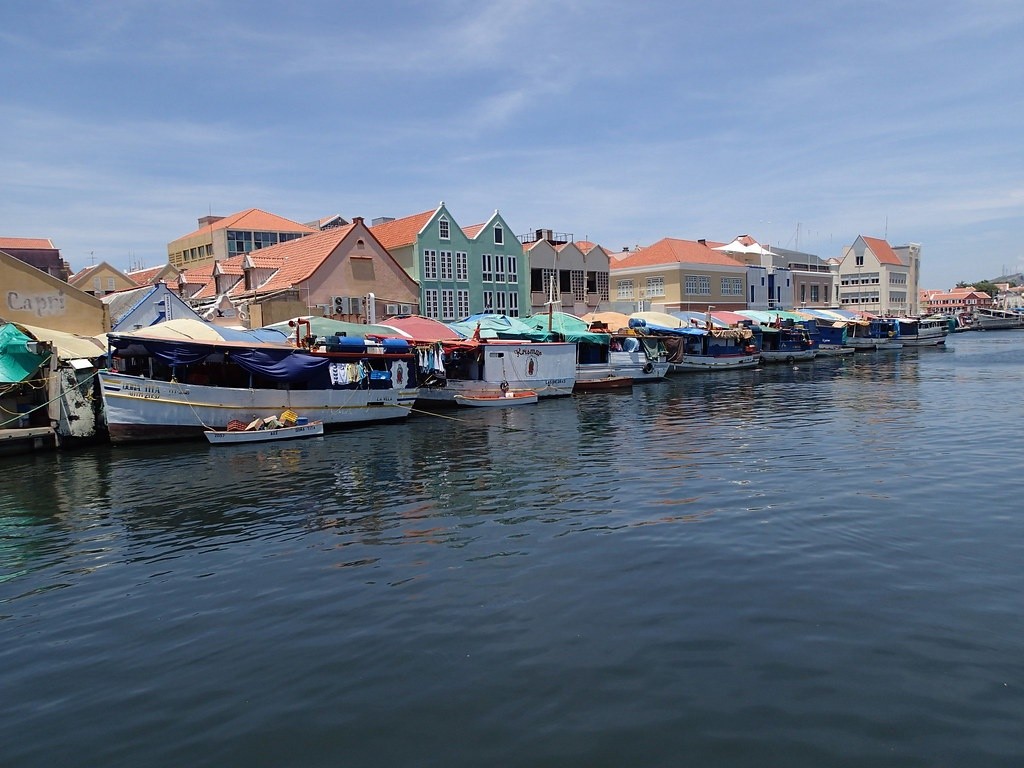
xmin=500 ymin=382 xmax=510 ymax=392
xmin=643 ymin=362 xmax=655 ymax=374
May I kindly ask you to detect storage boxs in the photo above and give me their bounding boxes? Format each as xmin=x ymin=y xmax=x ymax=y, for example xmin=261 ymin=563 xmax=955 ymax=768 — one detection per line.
xmin=226 ymin=409 xmax=308 ymax=430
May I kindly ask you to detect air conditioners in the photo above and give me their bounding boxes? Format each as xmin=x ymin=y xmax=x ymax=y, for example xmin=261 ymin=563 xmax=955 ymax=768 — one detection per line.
xmin=331 ymin=296 xmax=349 ymax=315
xmin=400 ymin=304 xmax=412 ymax=314
xmin=349 ymin=296 xmax=363 ymax=315
xmin=385 ymin=304 xmax=399 ymax=315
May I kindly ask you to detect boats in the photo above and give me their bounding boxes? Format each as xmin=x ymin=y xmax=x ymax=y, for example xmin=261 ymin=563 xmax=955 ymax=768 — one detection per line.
xmin=668 ymin=328 xmax=762 ymax=373
xmin=875 ymin=343 xmax=904 ymax=349
xmin=755 ymin=328 xmax=818 ymax=364
xmin=812 ymin=346 xmax=856 ymax=357
xmin=976 ymin=307 xmax=1022 ymax=330
xmin=845 ymin=319 xmax=950 ymax=349
xmin=454 ymin=391 xmax=538 ymax=407
xmin=574 ymin=376 xmax=634 ymax=390
xmin=574 ymin=347 xmax=672 ymax=381
xmin=98 ymin=318 xmax=422 ymax=445
xmin=202 ymin=419 xmax=325 ymax=443
xmin=417 ymin=341 xmax=577 ymax=400
